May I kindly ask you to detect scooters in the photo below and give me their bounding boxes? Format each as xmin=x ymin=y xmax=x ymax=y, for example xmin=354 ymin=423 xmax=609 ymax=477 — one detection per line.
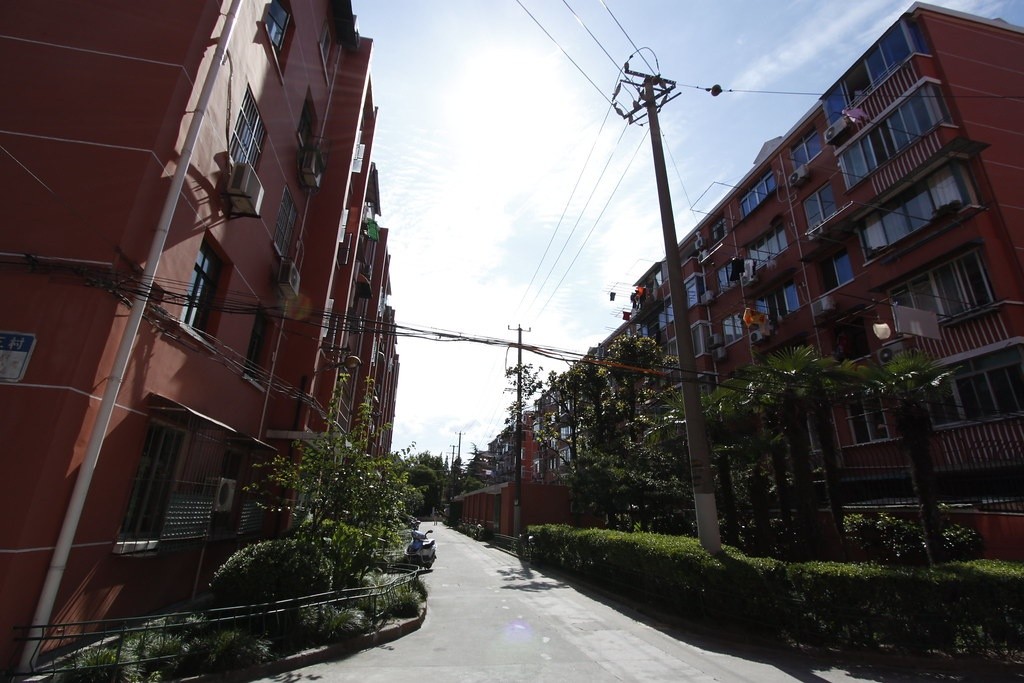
xmin=404 ymin=527 xmax=438 ymax=570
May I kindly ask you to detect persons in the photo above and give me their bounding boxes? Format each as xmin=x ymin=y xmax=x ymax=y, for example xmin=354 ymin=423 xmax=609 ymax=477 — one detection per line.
xmin=433 ymin=508 xmax=439 ymax=526
